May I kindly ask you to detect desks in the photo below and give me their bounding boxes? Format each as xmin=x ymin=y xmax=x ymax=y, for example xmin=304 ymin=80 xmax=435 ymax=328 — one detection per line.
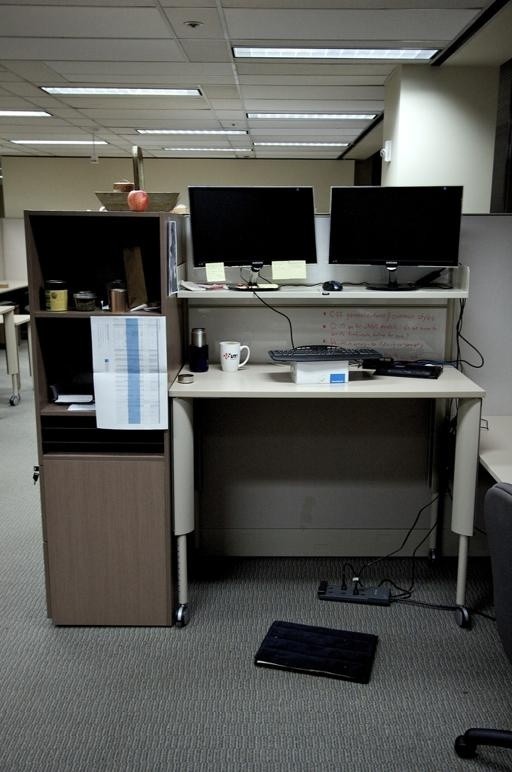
xmin=169 ymin=265 xmax=485 ymax=627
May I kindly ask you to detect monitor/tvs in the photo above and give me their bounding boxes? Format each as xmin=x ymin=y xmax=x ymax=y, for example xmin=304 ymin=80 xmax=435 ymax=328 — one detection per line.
xmin=328 ymin=185 xmax=464 ymax=291
xmin=188 ymin=184 xmax=316 ymax=289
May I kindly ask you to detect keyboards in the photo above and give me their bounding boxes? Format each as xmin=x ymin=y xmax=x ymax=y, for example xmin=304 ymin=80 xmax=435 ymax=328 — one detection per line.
xmin=268 ymin=348 xmax=382 ymax=362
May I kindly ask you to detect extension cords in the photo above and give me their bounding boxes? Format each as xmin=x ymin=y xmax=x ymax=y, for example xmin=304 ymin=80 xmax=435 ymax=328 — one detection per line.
xmin=318 ymin=585 xmax=393 ymax=607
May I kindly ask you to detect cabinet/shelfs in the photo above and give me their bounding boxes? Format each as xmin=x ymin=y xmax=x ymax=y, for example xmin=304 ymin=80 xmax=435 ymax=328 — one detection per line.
xmin=22 ymin=209 xmax=187 ymax=629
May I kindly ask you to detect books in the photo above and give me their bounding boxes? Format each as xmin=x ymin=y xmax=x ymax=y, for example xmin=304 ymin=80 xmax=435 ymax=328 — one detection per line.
xmin=51 ymin=384 xmax=93 ymax=404
xmin=254 ymin=619 xmax=380 ymax=685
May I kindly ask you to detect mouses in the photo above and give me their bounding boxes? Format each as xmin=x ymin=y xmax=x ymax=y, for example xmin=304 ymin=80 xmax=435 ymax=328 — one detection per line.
xmin=324 ymin=280 xmax=343 ymax=291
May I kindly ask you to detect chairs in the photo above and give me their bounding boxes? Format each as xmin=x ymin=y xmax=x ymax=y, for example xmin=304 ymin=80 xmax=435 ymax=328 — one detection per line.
xmin=454 ymin=482 xmax=511 ymax=761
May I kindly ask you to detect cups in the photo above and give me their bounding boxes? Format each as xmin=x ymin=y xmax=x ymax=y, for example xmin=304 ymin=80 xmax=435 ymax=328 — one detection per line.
xmin=74 ymin=292 xmax=97 ymax=311
xmin=219 ymin=341 xmax=250 ymax=371
xmin=187 ymin=328 xmax=211 ymax=372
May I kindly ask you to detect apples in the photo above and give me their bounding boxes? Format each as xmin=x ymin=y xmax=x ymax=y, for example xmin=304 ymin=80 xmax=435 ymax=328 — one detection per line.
xmin=128 ymin=189 xmax=148 ymax=212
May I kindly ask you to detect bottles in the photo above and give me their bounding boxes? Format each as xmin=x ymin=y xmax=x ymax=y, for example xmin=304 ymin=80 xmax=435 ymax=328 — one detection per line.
xmin=109 ymin=289 xmax=128 ymax=312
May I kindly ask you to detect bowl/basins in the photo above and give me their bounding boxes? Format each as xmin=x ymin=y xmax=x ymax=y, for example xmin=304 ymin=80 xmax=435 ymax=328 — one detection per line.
xmin=94 ymin=192 xmax=180 ymax=212
